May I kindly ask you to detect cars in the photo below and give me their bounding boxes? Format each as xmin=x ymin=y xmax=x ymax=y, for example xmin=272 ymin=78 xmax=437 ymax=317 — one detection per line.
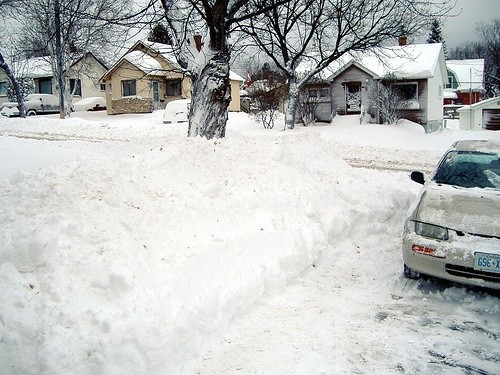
xmin=401 ymin=139 xmax=500 ymax=294
xmin=0 ymin=93 xmax=69 ymax=117
xmin=70 ymin=97 xmax=107 ymax=111
